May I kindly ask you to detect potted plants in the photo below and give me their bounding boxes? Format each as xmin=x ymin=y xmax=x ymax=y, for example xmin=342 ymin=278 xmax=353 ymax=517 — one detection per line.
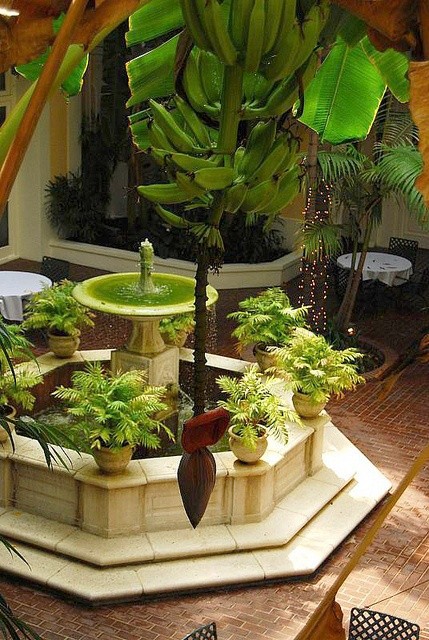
xmin=0 ymin=321 xmax=46 ymax=444
xmin=225 ymin=284 xmax=313 ymax=375
xmin=48 ymin=355 xmax=177 ymax=474
xmin=265 ymin=329 xmax=368 ymax=421
xmin=212 ymin=361 xmax=306 ymax=464
xmin=158 ymin=312 xmax=196 ymax=352
xmin=21 ymin=278 xmax=98 ymax=358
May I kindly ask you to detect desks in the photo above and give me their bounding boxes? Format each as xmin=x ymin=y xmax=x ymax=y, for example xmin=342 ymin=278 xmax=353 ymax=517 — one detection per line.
xmin=0 ymin=270 xmax=52 ymax=341
xmin=336 ymin=251 xmax=414 ymax=288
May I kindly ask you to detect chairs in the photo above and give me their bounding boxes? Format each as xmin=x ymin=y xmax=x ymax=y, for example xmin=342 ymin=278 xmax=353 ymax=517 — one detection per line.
xmin=38 ymin=255 xmax=71 ymax=283
xmin=333 ymin=264 xmax=380 ymax=321
xmin=387 ymin=236 xmax=419 ymax=270
xmin=181 ymin=620 xmax=219 ymax=640
xmin=345 ymin=607 xmax=421 ymax=640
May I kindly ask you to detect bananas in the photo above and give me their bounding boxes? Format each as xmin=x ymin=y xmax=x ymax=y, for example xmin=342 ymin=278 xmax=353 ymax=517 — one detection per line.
xmin=136 ymin=2 xmax=333 ymax=227
